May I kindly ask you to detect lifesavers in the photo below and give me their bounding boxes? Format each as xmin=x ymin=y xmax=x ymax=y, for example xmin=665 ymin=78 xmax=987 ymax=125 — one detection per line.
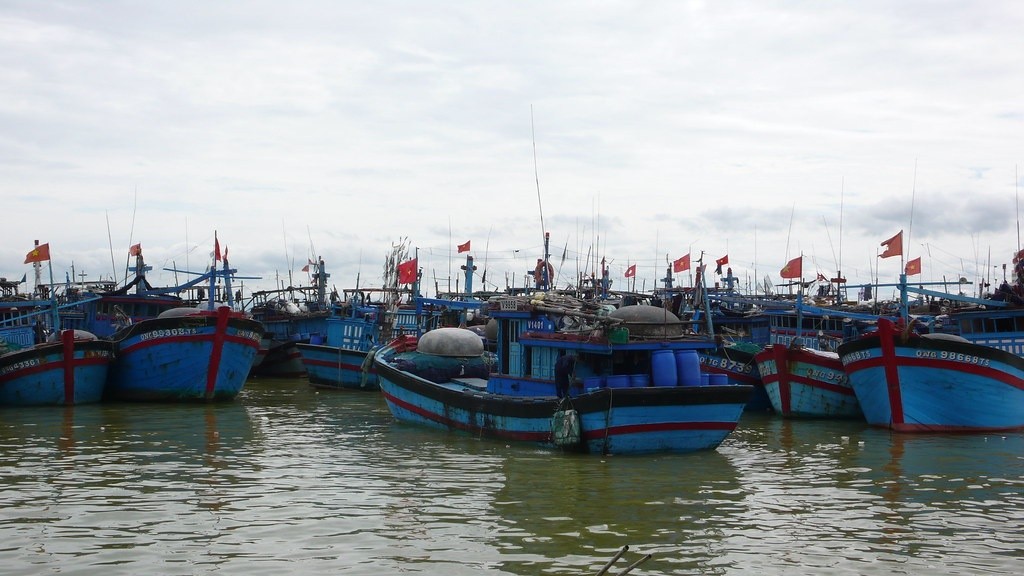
xmin=534 ymin=261 xmax=554 ymax=285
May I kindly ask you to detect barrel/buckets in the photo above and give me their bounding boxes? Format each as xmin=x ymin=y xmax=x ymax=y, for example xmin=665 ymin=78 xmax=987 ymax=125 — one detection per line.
xmin=700 ymin=374 xmax=709 ymax=385
xmin=709 ymin=374 xmax=727 ymax=385
xmin=651 ymin=350 xmax=677 ymax=387
xmin=673 ymin=350 xmax=700 ymax=385
xmin=583 ymin=377 xmax=601 ymax=393
xmin=605 ymin=375 xmax=629 ymax=387
xmin=630 ymin=374 xmax=650 ymax=387
xmin=310 ymin=332 xmax=322 ymax=345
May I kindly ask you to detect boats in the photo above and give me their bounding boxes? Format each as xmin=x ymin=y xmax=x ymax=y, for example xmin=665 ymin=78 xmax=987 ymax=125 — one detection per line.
xmin=755 ymin=269 xmax=1023 ymax=432
xmin=0 ymin=231 xmax=266 ymax=405
xmin=250 ymin=232 xmax=755 ymax=455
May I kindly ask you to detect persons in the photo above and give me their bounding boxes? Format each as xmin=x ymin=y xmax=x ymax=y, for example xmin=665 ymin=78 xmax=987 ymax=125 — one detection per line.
xmin=554 ymin=355 xmax=569 ymax=399
xmin=33 ymin=320 xmax=49 ymax=344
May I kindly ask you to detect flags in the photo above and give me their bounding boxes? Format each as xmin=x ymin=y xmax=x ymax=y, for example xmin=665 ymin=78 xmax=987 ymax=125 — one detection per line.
xmin=223 ymin=248 xmax=227 ymax=260
xmin=716 ymin=255 xmax=729 ymax=267
xmin=398 ymin=259 xmax=418 ymax=284
xmin=625 ymin=265 xmax=635 ymax=278
xmin=674 ymin=254 xmax=690 ymax=272
xmin=780 ymin=257 xmax=802 ymax=278
xmin=906 ymin=257 xmax=920 ymax=275
xmin=131 ymin=244 xmax=141 ymax=256
xmin=880 ymin=232 xmax=902 ymax=258
xmin=25 ymin=243 xmax=50 ymax=263
xmin=215 ymin=237 xmax=220 ymax=261
xmin=457 ymin=240 xmax=470 ymax=253
